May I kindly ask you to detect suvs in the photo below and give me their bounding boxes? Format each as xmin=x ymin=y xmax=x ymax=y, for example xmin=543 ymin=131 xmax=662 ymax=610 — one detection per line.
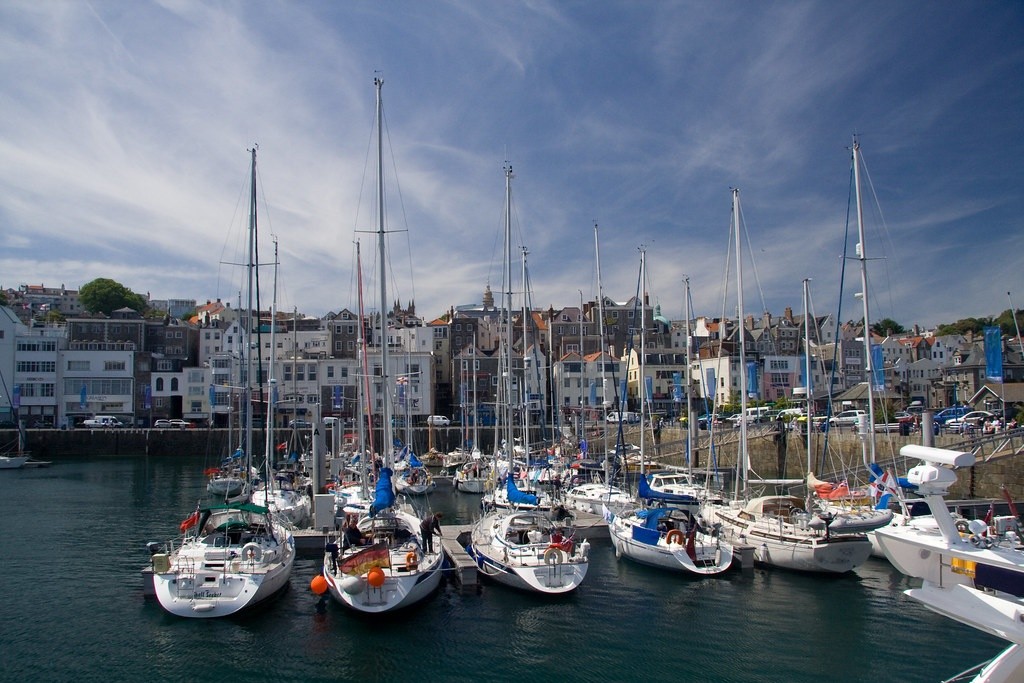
xmin=754 ymin=409 xmax=780 ymax=423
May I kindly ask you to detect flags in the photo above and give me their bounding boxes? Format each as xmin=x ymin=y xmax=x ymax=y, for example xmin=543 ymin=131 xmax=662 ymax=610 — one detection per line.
xmin=209 ymin=383 xmax=215 ymax=406
xmin=335 ymin=384 xmax=341 ymax=406
xmin=277 ymin=441 xmax=288 ymax=450
xmin=746 ymin=362 xmax=757 ymax=397
xmin=868 ymin=468 xmax=899 ymax=497
xmin=814 ymin=479 xmax=849 ymax=500
xmin=983 ymin=326 xmax=1003 ymax=383
xmin=589 ymin=373 xmax=682 ymax=407
xmin=868 ymin=343 xmax=885 ymax=391
xmin=80 ymin=383 xmax=87 ymax=409
xmin=146 ymin=385 xmax=151 ymax=408
xmin=800 ymin=354 xmax=813 ymax=389
xmin=13 ymin=385 xmax=19 ymax=409
xmin=705 ymin=368 xmax=716 ymax=400
xmin=180 ymin=501 xmax=201 ymax=530
xmin=395 ymin=376 xmax=408 ymax=386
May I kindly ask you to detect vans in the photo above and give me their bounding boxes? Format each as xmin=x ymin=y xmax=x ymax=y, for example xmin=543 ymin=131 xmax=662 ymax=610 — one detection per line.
xmin=775 ymin=409 xmax=802 ymax=421
xmin=933 ymin=405 xmax=976 ymax=426
xmin=828 ymin=409 xmax=867 ymax=427
xmin=94 ymin=415 xmax=124 ymax=428
xmin=324 ymin=417 xmax=347 ymax=427
xmin=427 ymin=416 xmax=451 ymax=427
xmin=606 ymin=411 xmax=635 ymax=425
xmin=738 ymin=404 xmax=773 ymax=424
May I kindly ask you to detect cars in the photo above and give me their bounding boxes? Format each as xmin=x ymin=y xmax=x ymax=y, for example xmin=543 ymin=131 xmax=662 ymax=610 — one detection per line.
xmin=392 ymin=419 xmax=405 ymax=427
xmin=289 ymin=419 xmax=312 ymax=428
xmin=84 ymin=419 xmax=107 ymax=429
xmin=726 ymin=413 xmax=740 ymax=421
xmin=945 ymin=410 xmax=1001 ymax=433
xmin=169 ymin=419 xmax=190 ymax=428
xmin=154 ymin=419 xmax=174 ymax=428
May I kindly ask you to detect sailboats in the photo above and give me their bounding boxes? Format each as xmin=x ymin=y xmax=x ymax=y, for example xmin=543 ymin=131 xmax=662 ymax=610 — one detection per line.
xmin=1 ymin=370 xmax=32 ymax=470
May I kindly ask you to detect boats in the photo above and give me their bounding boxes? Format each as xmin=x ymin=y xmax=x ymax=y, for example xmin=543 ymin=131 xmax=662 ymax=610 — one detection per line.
xmin=148 ymin=69 xmax=1023 ymax=683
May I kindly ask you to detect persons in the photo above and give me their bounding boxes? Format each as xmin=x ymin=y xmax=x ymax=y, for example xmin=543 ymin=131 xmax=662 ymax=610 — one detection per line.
xmin=961 ymin=415 xmax=1019 ymax=437
xmin=913 ymin=414 xmax=921 ymax=436
xmin=419 ymin=512 xmax=444 ymax=554
xmin=819 ymin=512 xmax=834 ymax=539
xmin=342 ymin=514 xmax=365 ymax=554
xmin=552 ymin=529 xmax=564 ymax=543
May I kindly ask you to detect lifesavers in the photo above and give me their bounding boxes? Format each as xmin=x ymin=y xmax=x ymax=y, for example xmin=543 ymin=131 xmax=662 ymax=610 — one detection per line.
xmin=544 ymin=547 xmax=563 ymax=565
xmin=665 ymin=528 xmax=684 ymax=546
xmin=241 ymin=541 xmax=262 ymax=562
xmin=405 ymin=551 xmax=419 ymax=571
